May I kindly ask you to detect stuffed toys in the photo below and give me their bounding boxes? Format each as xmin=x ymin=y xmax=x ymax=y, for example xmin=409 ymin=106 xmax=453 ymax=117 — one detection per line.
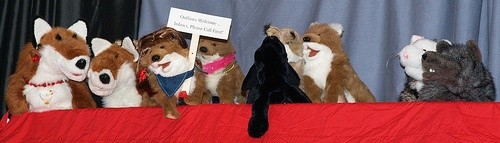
xmin=133 ymin=27 xmax=212 ymax=118
xmin=418 ymin=40 xmax=496 ymax=101
xmin=264 ymin=25 xmax=306 ymax=91
xmin=196 ymin=36 xmax=244 ymax=104
xmin=88 ymin=36 xmax=143 ymax=107
xmin=396 ymin=35 xmax=452 ymax=102
xmin=6 ymin=15 xmax=98 ymax=114
xmin=241 ymin=36 xmax=312 ymax=138
xmin=299 ymin=22 xmax=376 ymax=103
